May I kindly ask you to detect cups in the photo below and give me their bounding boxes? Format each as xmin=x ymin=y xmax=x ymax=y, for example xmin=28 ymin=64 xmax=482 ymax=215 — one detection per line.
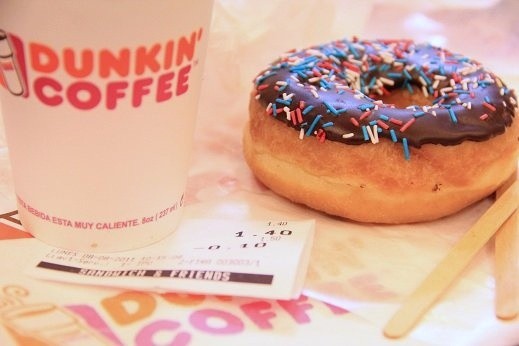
xmin=0 ymin=0 xmax=215 ymax=242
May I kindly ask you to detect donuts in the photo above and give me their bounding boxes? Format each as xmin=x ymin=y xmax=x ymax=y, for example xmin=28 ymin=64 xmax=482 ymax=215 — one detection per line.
xmin=242 ymin=34 xmax=517 ymax=227
